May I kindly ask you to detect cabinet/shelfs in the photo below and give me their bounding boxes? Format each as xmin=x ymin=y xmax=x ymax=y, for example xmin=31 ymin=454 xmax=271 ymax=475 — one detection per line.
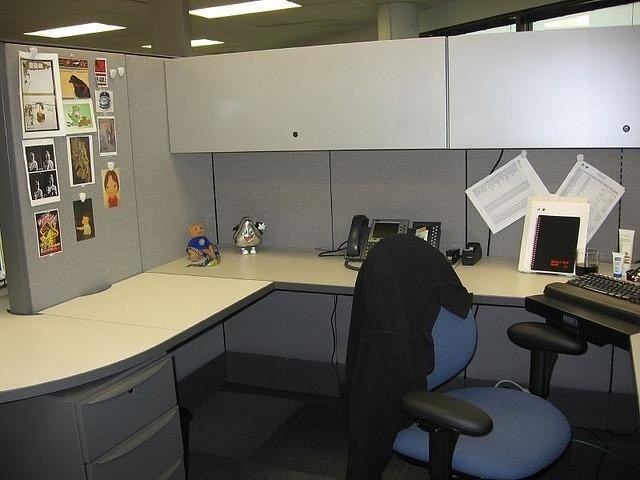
xmin=447 ymin=23 xmax=640 ymax=148
xmin=166 ymin=36 xmax=450 ymax=151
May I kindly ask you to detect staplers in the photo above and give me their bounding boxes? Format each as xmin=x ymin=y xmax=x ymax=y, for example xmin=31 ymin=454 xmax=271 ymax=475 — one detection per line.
xmin=446 ymin=249 xmax=462 ymax=269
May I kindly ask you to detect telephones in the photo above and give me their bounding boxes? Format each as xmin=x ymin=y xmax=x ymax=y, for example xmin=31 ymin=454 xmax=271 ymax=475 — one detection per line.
xmin=344 ymin=213 xmax=408 ymax=261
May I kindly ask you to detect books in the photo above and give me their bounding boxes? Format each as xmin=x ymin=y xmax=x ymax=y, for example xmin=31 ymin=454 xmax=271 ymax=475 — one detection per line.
xmin=531 ymin=214 xmax=580 ymax=273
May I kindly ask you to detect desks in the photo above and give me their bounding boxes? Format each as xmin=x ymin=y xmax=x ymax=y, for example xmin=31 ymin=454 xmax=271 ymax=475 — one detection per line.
xmin=0 ymin=246 xmax=640 ymax=480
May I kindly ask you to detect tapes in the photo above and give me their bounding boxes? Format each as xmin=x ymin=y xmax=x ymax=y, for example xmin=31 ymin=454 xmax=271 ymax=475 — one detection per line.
xmin=464 ymin=247 xmax=474 ymax=251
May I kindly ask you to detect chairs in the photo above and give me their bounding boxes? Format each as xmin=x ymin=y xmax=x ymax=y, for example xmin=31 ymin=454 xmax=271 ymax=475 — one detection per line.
xmin=347 ymin=233 xmax=588 ymax=480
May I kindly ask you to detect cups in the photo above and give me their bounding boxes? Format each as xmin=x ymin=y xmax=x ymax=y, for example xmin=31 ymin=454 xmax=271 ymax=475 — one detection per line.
xmin=576 ymin=248 xmax=599 ymax=276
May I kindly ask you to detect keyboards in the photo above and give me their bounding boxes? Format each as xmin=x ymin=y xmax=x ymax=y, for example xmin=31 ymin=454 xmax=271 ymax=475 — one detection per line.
xmin=544 ymin=273 xmax=640 ymax=322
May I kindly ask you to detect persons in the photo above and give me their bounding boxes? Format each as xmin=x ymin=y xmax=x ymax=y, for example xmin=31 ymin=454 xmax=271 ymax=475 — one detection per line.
xmin=28 ymin=151 xmax=57 ymax=200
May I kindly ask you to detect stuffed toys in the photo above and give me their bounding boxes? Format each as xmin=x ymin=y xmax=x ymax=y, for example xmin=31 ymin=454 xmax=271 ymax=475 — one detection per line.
xmin=186 ymin=225 xmax=220 ymax=267
